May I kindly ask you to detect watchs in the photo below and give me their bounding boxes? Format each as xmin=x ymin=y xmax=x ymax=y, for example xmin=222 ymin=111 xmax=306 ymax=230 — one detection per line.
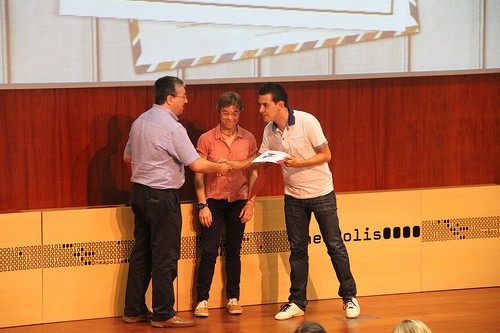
xmin=198 ymin=203 xmax=208 ymax=209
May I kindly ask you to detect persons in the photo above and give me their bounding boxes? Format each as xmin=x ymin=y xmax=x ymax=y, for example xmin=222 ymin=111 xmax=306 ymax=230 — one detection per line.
xmin=195 ymin=92 xmax=258 ymax=317
xmin=294 ymin=321 xmax=327 ymax=333
xmin=216 ymin=83 xmax=361 ymax=320
xmin=392 ymin=319 xmax=433 ymax=333
xmin=123 ymin=76 xmax=232 ymax=327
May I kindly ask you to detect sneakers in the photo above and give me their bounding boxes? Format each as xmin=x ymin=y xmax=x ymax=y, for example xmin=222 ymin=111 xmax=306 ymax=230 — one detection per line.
xmin=274 ymin=302 xmax=304 ymax=320
xmin=226 ymin=299 xmax=242 ymax=313
xmin=195 ymin=300 xmax=209 ymax=316
xmin=343 ymin=297 xmax=360 ymax=317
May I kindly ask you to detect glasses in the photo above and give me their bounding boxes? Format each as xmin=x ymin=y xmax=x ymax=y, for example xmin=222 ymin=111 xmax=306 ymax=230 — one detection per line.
xmin=168 ymin=94 xmax=186 ymax=99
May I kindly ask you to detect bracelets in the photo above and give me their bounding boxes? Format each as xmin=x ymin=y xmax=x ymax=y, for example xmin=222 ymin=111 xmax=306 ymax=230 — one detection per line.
xmin=246 ymin=203 xmax=254 ymax=208
xmin=250 ymin=200 xmax=254 ymax=202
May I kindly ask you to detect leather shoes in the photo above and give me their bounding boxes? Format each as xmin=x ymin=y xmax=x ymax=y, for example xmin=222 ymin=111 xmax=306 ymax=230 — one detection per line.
xmin=151 ymin=315 xmax=196 ymax=327
xmin=123 ymin=311 xmax=153 ymax=322
